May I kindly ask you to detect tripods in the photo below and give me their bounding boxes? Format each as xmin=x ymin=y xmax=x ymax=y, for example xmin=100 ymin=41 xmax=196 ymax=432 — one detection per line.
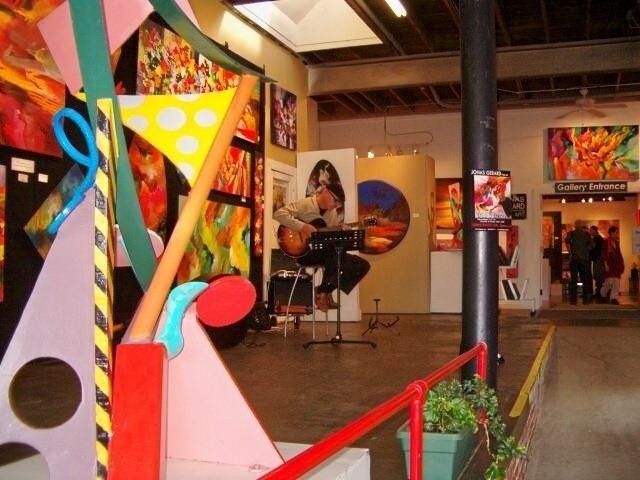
xmin=302 ymin=255 xmax=375 ymax=349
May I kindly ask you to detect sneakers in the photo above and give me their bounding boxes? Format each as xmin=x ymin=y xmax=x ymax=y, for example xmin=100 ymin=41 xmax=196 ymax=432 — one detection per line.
xmin=568 ymin=296 xmax=621 ymax=305
xmin=314 ymin=285 xmax=329 ymax=313
xmin=327 ymin=292 xmax=339 ymax=311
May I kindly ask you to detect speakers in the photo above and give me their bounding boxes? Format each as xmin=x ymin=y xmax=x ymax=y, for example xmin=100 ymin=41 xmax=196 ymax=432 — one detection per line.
xmin=268 ymin=277 xmax=312 ymax=315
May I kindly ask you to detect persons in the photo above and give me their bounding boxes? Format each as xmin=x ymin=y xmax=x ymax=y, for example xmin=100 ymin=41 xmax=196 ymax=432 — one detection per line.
xmin=271 ymin=184 xmax=370 ymax=313
xmin=563 ymin=226 xmax=624 ymax=304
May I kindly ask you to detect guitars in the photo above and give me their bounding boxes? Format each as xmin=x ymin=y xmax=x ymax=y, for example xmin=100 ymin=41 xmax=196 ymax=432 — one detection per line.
xmin=278 ymin=216 xmax=378 ymax=258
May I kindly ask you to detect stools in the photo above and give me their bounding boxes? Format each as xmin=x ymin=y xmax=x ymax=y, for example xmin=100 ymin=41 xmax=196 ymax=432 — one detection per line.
xmin=283 ymin=256 xmax=329 ymax=341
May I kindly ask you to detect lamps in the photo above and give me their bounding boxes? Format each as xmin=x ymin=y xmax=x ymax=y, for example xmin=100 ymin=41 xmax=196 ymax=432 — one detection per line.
xmin=385 ymin=0 xmax=411 ymax=21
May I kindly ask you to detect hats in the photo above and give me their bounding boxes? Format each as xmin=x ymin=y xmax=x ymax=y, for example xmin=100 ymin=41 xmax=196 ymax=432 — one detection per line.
xmin=326 ymin=183 xmax=346 ymax=203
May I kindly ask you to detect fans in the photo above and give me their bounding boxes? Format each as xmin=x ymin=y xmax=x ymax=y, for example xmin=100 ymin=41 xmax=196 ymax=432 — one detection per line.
xmin=551 ymin=89 xmax=629 ymax=120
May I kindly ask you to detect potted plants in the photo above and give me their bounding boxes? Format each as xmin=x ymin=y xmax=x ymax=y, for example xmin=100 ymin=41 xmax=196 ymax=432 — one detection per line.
xmin=396 ymin=380 xmax=528 ymax=480
